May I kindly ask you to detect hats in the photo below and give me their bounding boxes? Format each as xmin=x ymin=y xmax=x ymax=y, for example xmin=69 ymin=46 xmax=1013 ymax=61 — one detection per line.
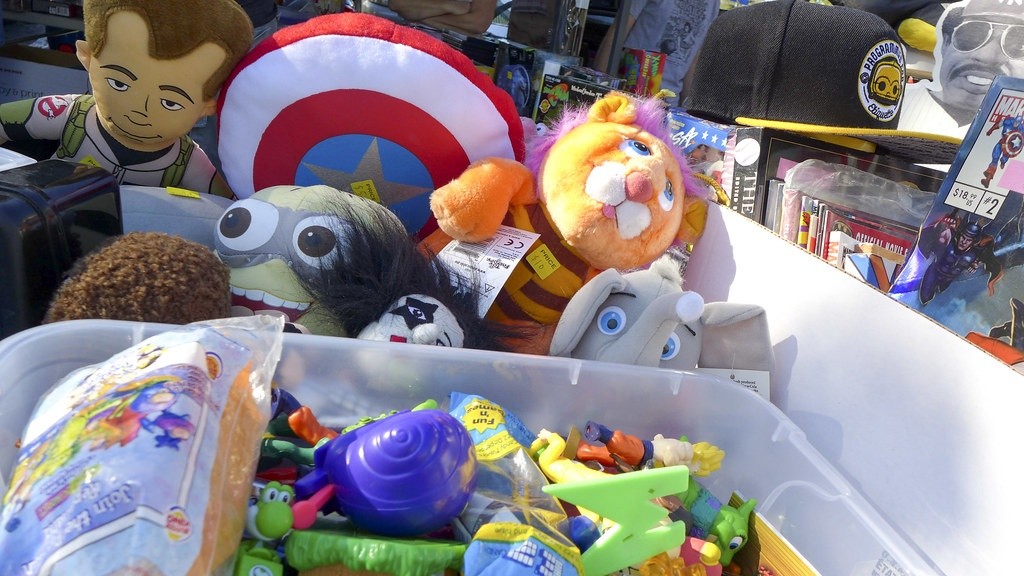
xmin=961 ymin=0 xmax=1024 ymax=24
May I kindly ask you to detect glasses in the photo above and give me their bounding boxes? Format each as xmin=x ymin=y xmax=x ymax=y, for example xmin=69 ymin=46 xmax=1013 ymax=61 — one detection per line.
xmin=951 ymin=19 xmax=1024 ymax=63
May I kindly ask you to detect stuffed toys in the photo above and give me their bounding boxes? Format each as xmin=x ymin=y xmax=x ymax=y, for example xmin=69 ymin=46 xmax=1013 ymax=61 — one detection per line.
xmin=2 ymin=1 xmax=534 ymax=393
xmin=548 ymin=252 xmax=780 ymax=408
xmin=430 ymin=84 xmax=730 ymax=357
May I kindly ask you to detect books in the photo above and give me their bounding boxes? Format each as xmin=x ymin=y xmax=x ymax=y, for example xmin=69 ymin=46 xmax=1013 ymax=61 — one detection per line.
xmin=767 ymin=176 xmax=923 ymax=296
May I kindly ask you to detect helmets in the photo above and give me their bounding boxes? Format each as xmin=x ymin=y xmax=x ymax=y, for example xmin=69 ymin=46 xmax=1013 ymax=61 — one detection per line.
xmin=961 ymin=216 xmax=982 ymax=241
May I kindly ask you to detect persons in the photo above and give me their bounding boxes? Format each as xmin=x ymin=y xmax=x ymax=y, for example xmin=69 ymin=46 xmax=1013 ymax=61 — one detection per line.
xmin=896 ymin=0 xmax=1024 ymax=173
xmin=594 ymin=0 xmax=723 ymax=111
